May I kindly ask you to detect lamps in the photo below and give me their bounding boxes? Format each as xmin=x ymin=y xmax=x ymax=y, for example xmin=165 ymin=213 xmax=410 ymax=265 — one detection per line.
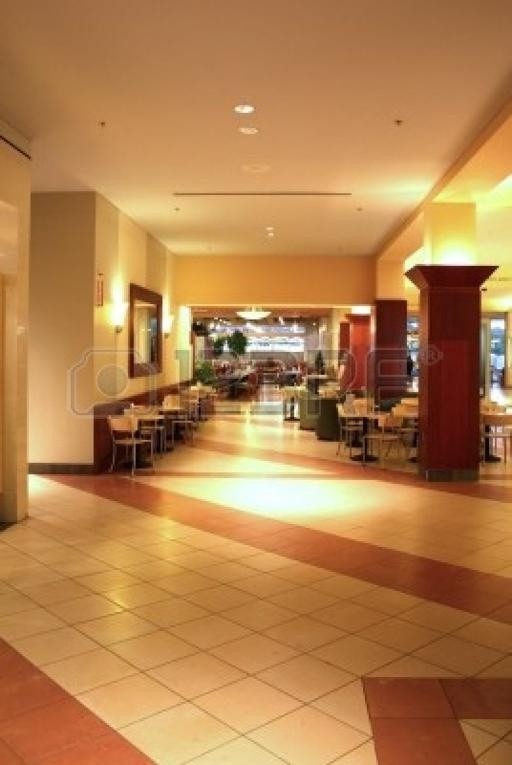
xmin=235 ymin=303 xmax=271 ymax=321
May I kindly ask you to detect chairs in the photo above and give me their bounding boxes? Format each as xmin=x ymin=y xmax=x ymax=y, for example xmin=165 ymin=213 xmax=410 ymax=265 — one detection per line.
xmin=479 ymin=399 xmax=512 ymax=463
xmin=109 ymin=380 xmax=216 ymax=477
xmin=298 ymin=389 xmax=419 ymax=471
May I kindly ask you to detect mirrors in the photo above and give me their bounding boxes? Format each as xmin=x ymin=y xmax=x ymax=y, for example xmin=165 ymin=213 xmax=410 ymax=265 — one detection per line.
xmin=127 ymin=283 xmax=162 ymax=378
